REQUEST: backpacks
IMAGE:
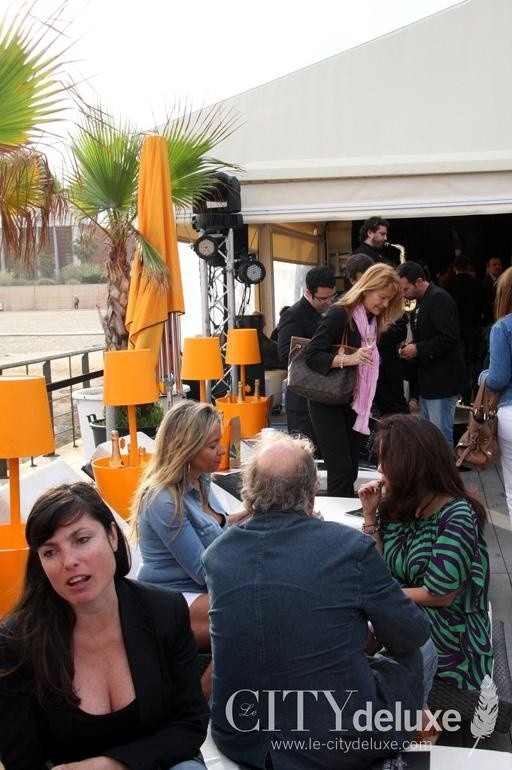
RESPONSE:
[285,334,362,406]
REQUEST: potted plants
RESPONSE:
[61,82,246,448]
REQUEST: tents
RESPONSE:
[124,136,186,396]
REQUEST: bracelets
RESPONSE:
[340,353,349,371]
[360,516,381,535]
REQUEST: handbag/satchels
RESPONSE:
[455,373,501,470]
[286,305,358,405]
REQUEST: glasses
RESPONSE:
[316,294,338,303]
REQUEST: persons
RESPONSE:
[0,478,212,770]
[197,428,440,769]
[443,255,485,416]
[476,264,512,536]
[483,255,504,325]
[304,261,404,498]
[131,397,232,704]
[346,254,410,415]
[270,306,289,343]
[277,265,336,459]
[357,216,390,264]
[395,262,459,460]
[250,311,277,358]
[357,412,495,689]
